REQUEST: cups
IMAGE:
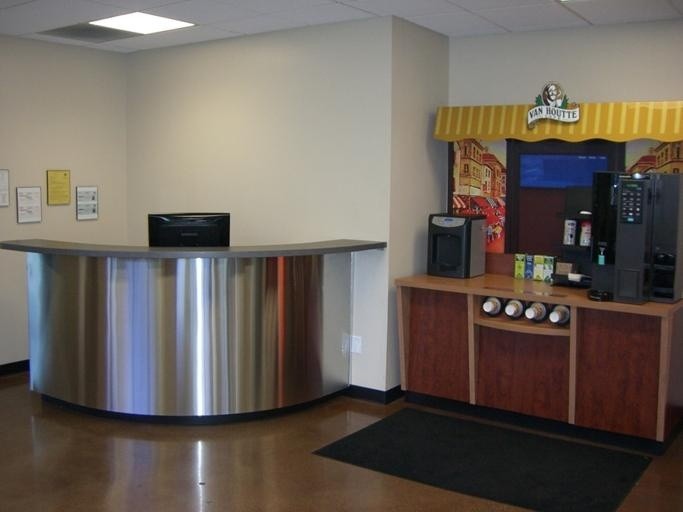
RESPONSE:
[483,298,570,326]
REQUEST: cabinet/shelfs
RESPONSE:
[395,271,682,444]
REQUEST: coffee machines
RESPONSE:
[428,214,489,277]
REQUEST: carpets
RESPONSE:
[309,406,654,512]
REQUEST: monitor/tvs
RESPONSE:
[148,214,232,247]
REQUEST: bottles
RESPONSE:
[578,219,591,247]
[563,218,576,246]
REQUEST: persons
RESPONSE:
[544,84,560,108]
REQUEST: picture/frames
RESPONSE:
[15,187,41,224]
[75,186,97,220]
[46,170,70,205]
[0,168,9,207]
